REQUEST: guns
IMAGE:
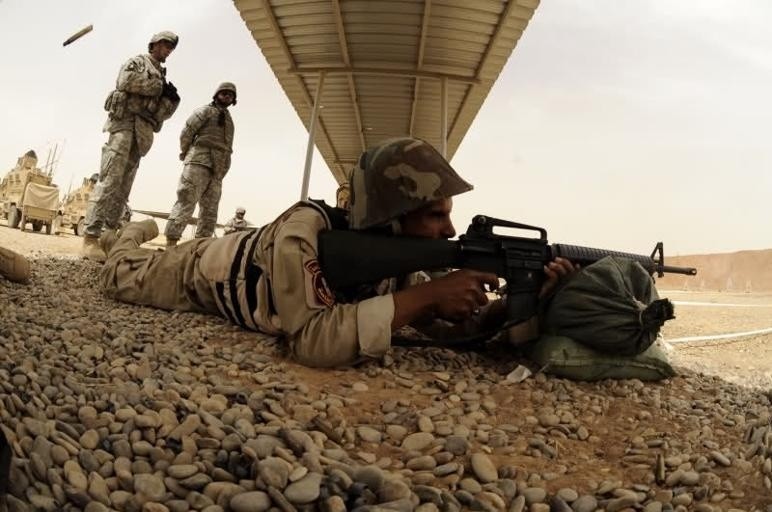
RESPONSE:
[313,213,698,325]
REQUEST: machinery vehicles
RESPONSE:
[62,174,130,237]
[1,150,60,233]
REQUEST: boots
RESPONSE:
[101,219,158,252]
[79,232,107,262]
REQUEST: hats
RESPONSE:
[236,207,245,214]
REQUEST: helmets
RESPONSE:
[147,30,179,53]
[349,137,473,231]
[213,82,236,99]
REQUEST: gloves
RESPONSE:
[179,152,185,161]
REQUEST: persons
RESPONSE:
[78,28,182,263]
[223,208,247,235]
[336,168,352,209]
[163,81,238,249]
[97,136,581,371]
